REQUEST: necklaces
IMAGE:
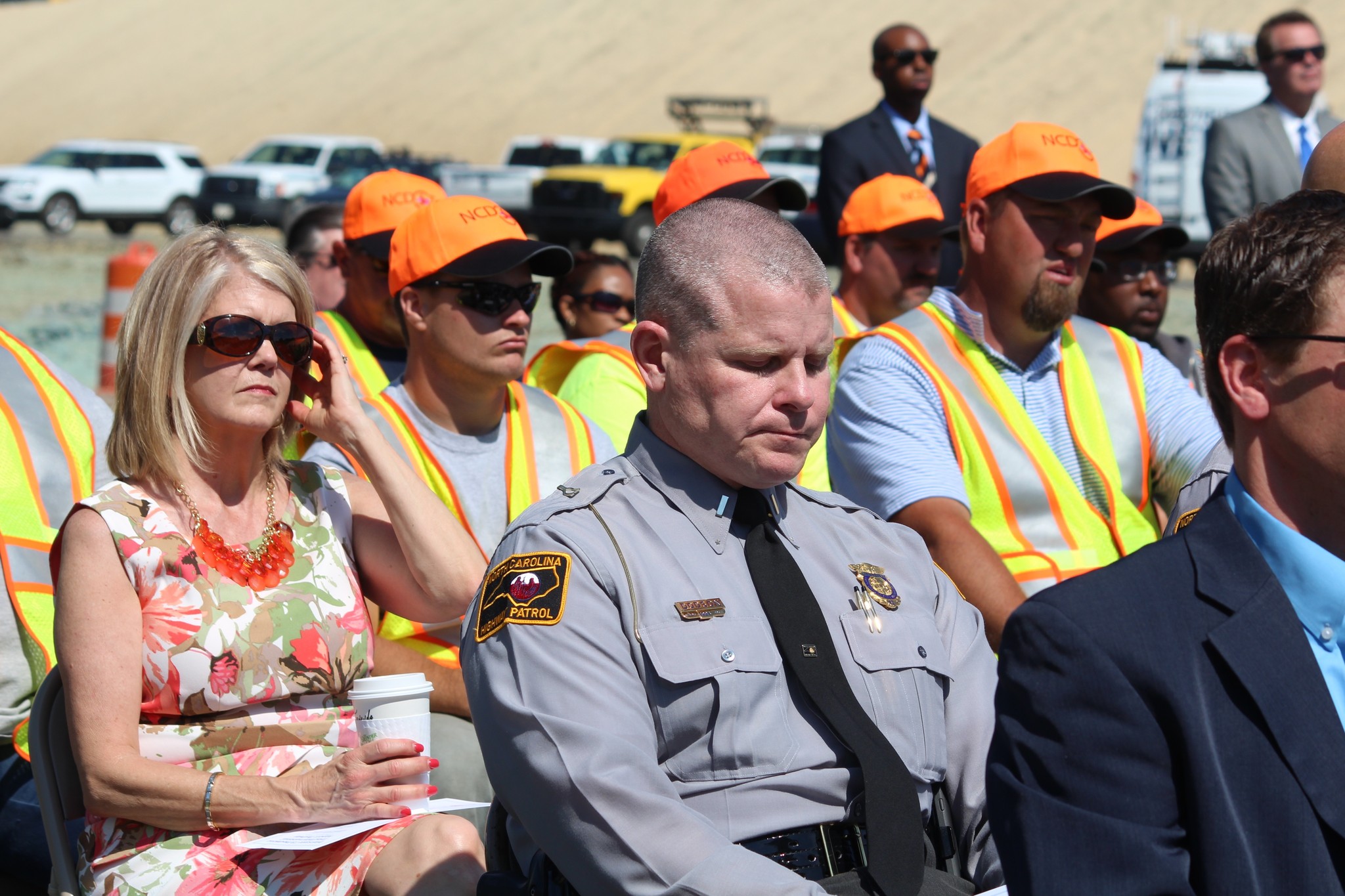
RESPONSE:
[165,458,295,591]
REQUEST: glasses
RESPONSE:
[886,50,936,67]
[352,242,388,270]
[1108,257,1177,283]
[422,277,541,316]
[189,315,315,363]
[1268,46,1323,64]
[577,291,635,316]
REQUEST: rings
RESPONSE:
[341,355,348,364]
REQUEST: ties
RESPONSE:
[736,488,924,896]
[907,129,937,191]
[1297,124,1312,169]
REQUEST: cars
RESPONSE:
[757,133,824,224]
[278,160,437,236]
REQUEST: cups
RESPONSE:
[347,673,436,814]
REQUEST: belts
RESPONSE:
[742,822,940,882]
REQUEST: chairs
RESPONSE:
[27,662,86,896]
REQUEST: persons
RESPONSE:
[48,227,488,896]
[459,197,999,896]
[284,9,1205,846]
[985,189,1345,896]
[1,333,120,896]
[1202,10,1345,235]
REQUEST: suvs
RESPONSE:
[531,131,756,257]
[198,132,389,229]
[1,141,208,237]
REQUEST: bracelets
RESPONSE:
[203,771,227,832]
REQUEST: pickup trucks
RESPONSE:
[432,138,606,235]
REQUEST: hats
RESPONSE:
[965,120,1136,220]
[1094,196,1189,256]
[343,166,447,248]
[837,172,957,239]
[387,194,573,298]
[651,141,807,227]
